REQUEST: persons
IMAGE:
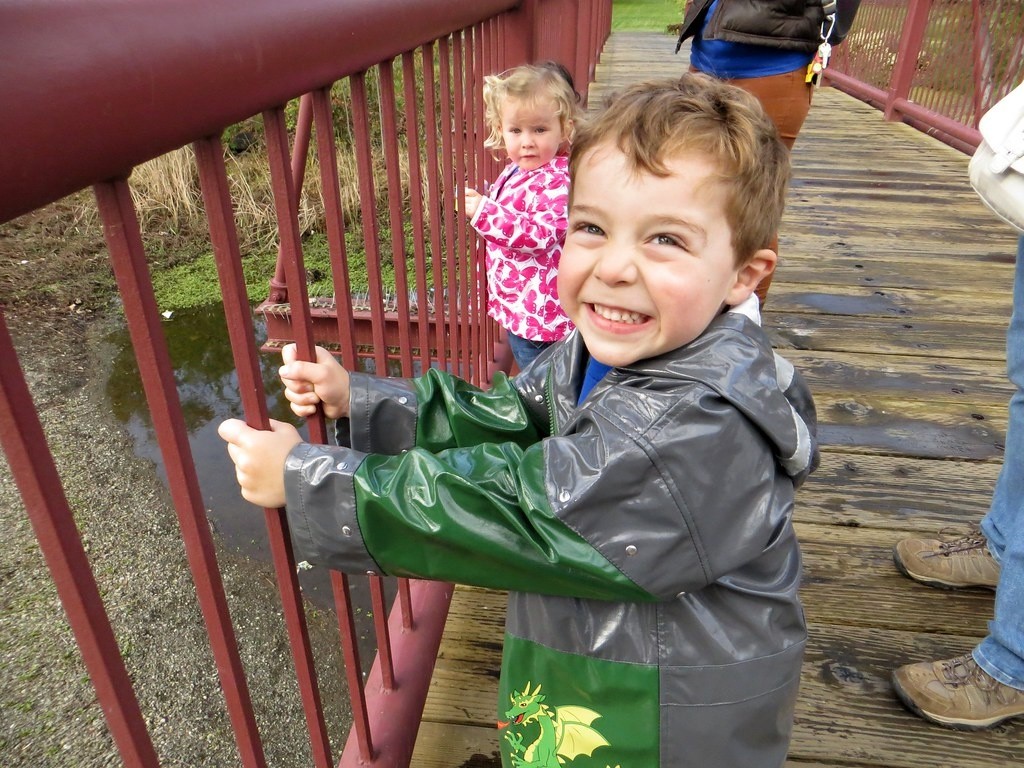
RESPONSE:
[455,63,574,371]
[675,0,861,314]
[890,81,1024,732]
[218,72,819,768]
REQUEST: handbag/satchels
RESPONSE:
[968,80,1024,233]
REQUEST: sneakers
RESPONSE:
[890,653,1024,732]
[893,521,1001,593]
